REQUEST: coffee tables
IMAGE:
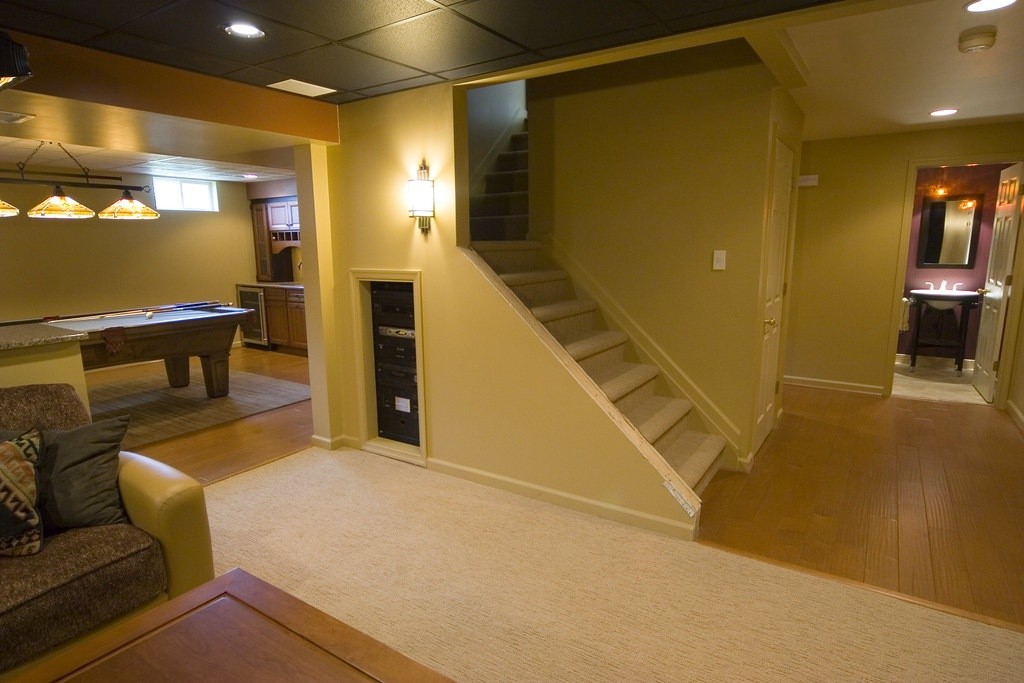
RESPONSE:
[1,567,457,683]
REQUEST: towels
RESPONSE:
[899,297,910,331]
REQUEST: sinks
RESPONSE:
[910,289,979,309]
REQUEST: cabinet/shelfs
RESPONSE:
[908,294,979,377]
[268,201,300,231]
[250,203,295,283]
[285,289,308,358]
[264,288,289,355]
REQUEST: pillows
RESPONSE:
[0,414,131,530]
[0,428,44,558]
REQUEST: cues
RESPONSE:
[48,299,233,324]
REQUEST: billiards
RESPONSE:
[146,311,154,318]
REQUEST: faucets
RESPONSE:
[939,280,947,290]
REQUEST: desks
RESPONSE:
[0,299,255,399]
[0,322,93,424]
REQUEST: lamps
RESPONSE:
[402,159,436,237]
[0,141,161,219]
[932,168,948,197]
[964,167,974,207]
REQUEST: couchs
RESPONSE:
[0,383,214,674]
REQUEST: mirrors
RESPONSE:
[915,193,985,270]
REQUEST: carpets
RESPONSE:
[85,362,312,452]
[204,447,1024,683]
